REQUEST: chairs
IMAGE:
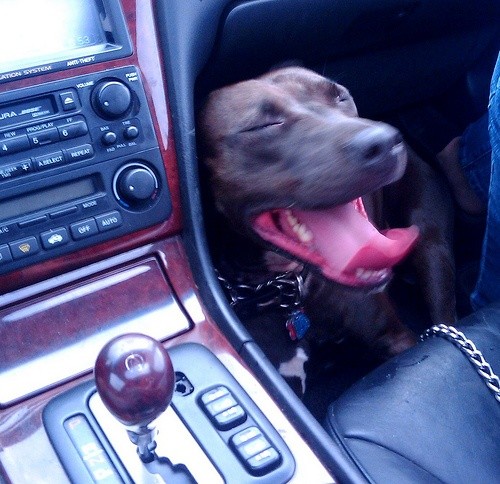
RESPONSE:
[330,303,499,484]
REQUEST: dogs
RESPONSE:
[196,66,465,358]
